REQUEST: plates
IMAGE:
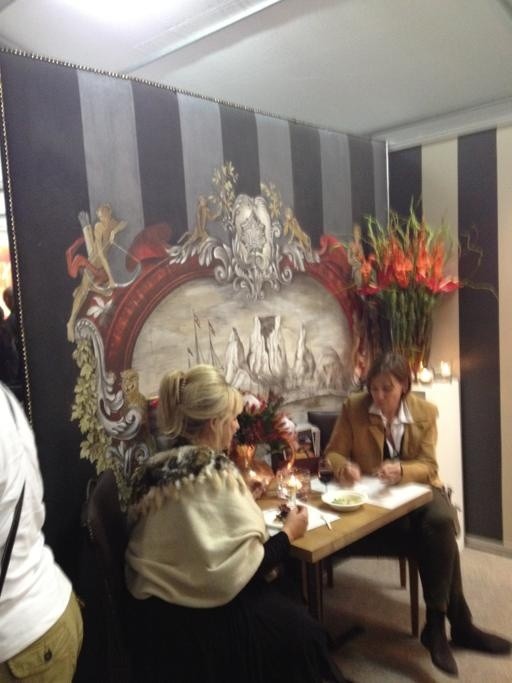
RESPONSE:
[262,506,320,528]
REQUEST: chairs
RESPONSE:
[307,409,418,637]
[78,467,129,683]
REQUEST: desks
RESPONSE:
[254,466,434,640]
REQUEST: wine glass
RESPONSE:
[318,458,334,491]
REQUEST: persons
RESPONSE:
[325,353,512,678]
[0,382,84,683]
[122,364,354,683]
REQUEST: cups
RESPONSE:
[275,468,311,499]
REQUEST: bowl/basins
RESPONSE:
[321,490,369,512]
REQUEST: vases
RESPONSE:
[230,444,275,500]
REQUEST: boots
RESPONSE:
[449,609,510,653]
[421,615,458,676]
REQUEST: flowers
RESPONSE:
[326,195,498,389]
[223,388,295,475]
